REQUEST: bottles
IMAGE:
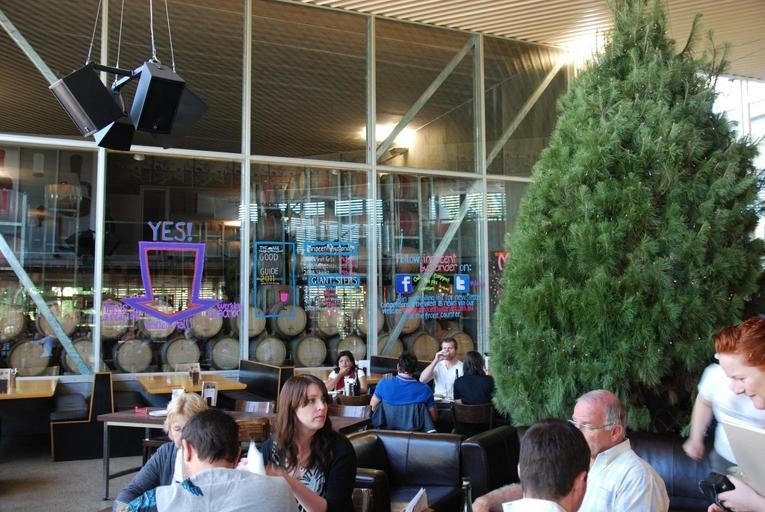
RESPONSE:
[189,365,199,386]
[0,372,7,394]
[461,482,472,512]
[345,381,359,396]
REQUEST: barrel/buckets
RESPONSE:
[0,301,476,375]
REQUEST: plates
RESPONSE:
[149,410,169,416]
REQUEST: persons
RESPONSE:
[111,392,210,512]
[705,316,765,512]
[237,374,358,512]
[369,351,439,423]
[125,409,301,512]
[503,418,592,511]
[418,337,464,402]
[682,360,764,476]
[471,390,671,512]
[325,350,368,395]
[453,351,495,405]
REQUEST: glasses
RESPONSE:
[567,420,613,431]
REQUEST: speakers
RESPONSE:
[130,61,187,136]
[93,86,136,152]
[47,63,124,140]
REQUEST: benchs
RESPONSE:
[0,355,509,512]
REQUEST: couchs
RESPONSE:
[459,422,737,512]
